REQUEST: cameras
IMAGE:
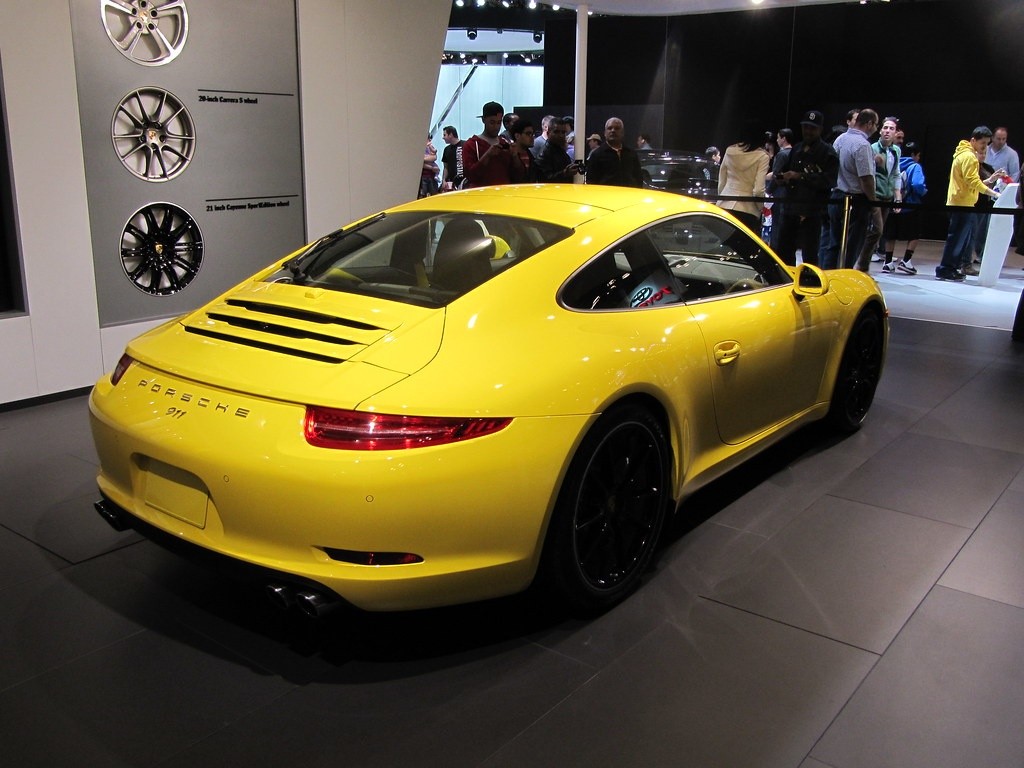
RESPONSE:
[775,172,784,179]
[574,160,585,175]
[499,142,510,149]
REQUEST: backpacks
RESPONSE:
[900,163,917,199]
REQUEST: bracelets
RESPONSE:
[987,178,993,183]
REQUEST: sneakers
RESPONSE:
[935,265,966,282]
[881,260,895,273]
[870,254,880,262]
[876,251,898,262]
[897,257,917,275]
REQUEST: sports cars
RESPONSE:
[88,183,889,615]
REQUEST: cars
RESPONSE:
[636,149,775,227]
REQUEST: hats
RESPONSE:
[586,133,601,141]
[800,110,824,129]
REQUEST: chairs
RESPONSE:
[429,217,492,291]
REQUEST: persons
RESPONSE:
[821,108,879,269]
[587,117,643,188]
[637,133,652,150]
[717,118,770,239]
[588,134,601,161]
[418,101,578,199]
[935,127,1024,282]
[772,110,840,265]
[831,106,1024,354]
[857,116,927,274]
[705,146,721,165]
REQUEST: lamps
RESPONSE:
[533,31,542,43]
[467,28,477,40]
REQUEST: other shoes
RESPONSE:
[961,265,980,276]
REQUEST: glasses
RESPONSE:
[553,129,567,135]
[521,131,536,138]
